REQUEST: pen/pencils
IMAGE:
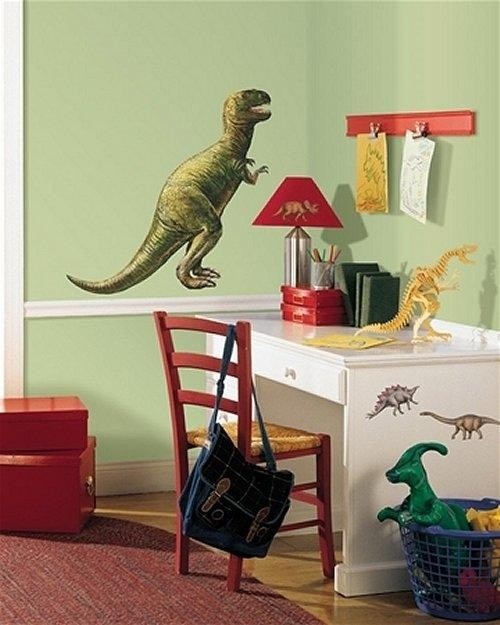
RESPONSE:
[305,243,342,287]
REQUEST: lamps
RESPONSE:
[252,175,343,287]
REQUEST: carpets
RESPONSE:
[1,516,326,625]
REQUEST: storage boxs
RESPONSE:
[0,397,96,536]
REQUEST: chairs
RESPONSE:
[150,309,332,591]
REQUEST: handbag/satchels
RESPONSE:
[177,327,295,558]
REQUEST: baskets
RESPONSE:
[396,498,499,620]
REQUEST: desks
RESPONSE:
[196,311,500,596]
